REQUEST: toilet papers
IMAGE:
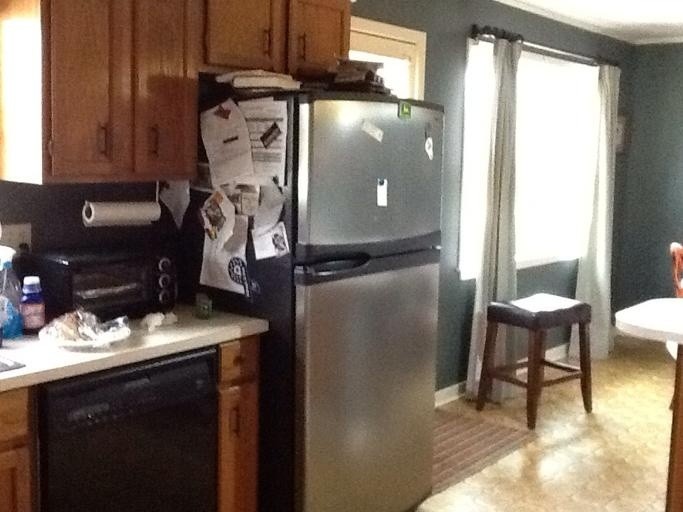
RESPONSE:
[82,201,162,228]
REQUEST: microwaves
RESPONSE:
[20,244,177,326]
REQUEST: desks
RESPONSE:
[615,297,683,511]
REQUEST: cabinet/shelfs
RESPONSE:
[0,0,202,185]
[202,0,347,78]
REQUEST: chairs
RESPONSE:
[669,242,683,299]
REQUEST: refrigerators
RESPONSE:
[194,87,447,511]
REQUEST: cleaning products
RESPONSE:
[0,245,24,339]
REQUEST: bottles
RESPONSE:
[19,276,45,337]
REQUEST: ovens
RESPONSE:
[36,349,218,511]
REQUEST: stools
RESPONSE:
[476,291,592,431]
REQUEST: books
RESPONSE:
[231,75,303,91]
[214,69,293,85]
[323,59,391,95]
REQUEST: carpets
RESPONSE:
[431,406,540,496]
